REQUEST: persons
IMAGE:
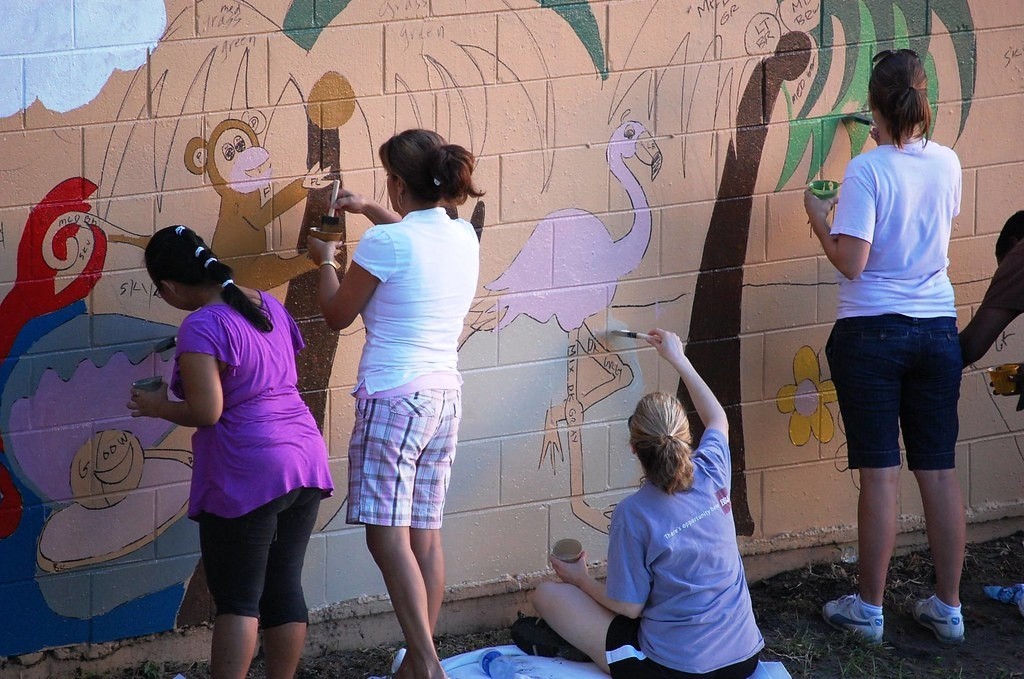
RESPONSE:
[531,328,765,679]
[127,225,335,678]
[306,129,486,679]
[804,49,966,649]
[957,210,1024,411]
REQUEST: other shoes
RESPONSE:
[511,616,593,662]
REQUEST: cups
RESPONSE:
[309,226,343,242]
[550,538,583,563]
[131,376,163,392]
[987,364,1019,394]
[807,180,840,199]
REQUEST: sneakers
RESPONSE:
[821,593,884,642]
[912,593,965,645]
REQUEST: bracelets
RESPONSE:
[318,260,337,270]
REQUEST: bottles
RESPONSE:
[478,648,516,679]
[983,585,1022,606]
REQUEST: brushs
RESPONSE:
[320,180,341,232]
[155,337,178,352]
[611,329,661,341]
[846,113,878,127]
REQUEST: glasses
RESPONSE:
[866,48,921,101]
